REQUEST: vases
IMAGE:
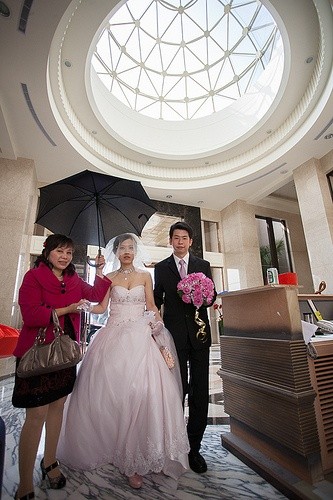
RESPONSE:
[218,320,224,335]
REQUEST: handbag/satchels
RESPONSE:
[17,310,83,378]
[158,335,175,369]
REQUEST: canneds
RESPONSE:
[267,268,279,285]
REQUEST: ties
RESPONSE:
[179,260,186,279]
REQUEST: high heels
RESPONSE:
[14,490,35,500]
[40,457,67,489]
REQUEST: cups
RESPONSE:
[302,313,314,325]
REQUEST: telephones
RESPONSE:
[317,319,333,333]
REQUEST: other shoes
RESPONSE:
[129,474,143,489]
[188,450,208,473]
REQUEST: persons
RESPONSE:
[56,232,191,489]
[11,234,112,500]
[152,221,218,473]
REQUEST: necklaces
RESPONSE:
[57,275,63,278]
[117,266,135,280]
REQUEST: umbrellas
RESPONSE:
[33,170,157,268]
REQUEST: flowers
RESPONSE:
[176,272,214,307]
[213,302,223,320]
